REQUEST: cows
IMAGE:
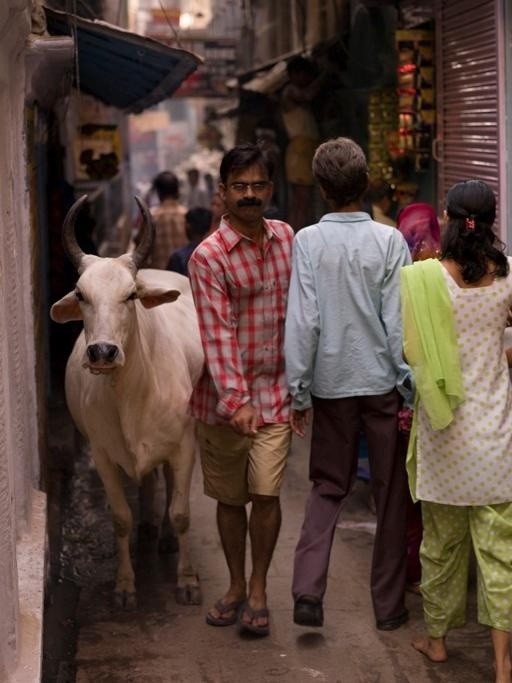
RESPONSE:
[50,193,205,611]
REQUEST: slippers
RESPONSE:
[207,599,323,635]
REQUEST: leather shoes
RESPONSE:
[377,613,408,630]
